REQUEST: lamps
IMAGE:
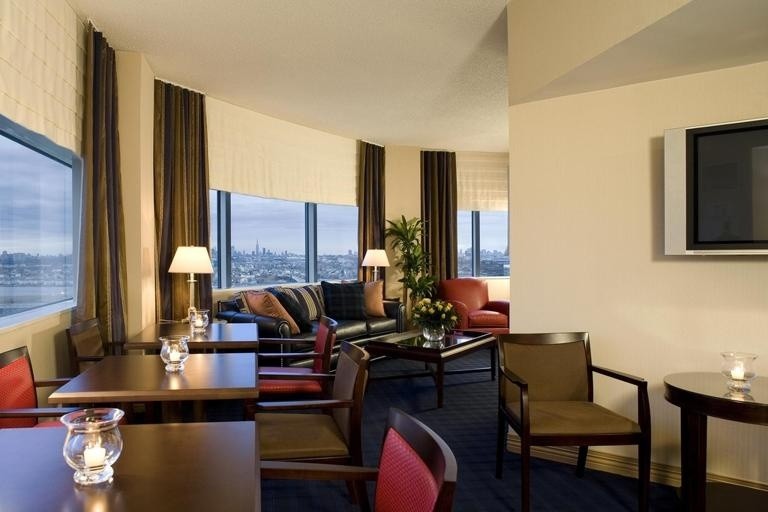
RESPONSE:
[167,246,215,322]
[361,249,390,281]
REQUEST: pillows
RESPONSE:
[233,281,385,333]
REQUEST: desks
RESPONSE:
[663,373,768,512]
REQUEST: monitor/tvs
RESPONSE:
[664,117,768,256]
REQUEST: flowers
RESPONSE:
[412,298,462,329]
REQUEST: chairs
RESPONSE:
[437,278,510,341]
[497,332,652,512]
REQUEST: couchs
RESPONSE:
[218,281,408,374]
